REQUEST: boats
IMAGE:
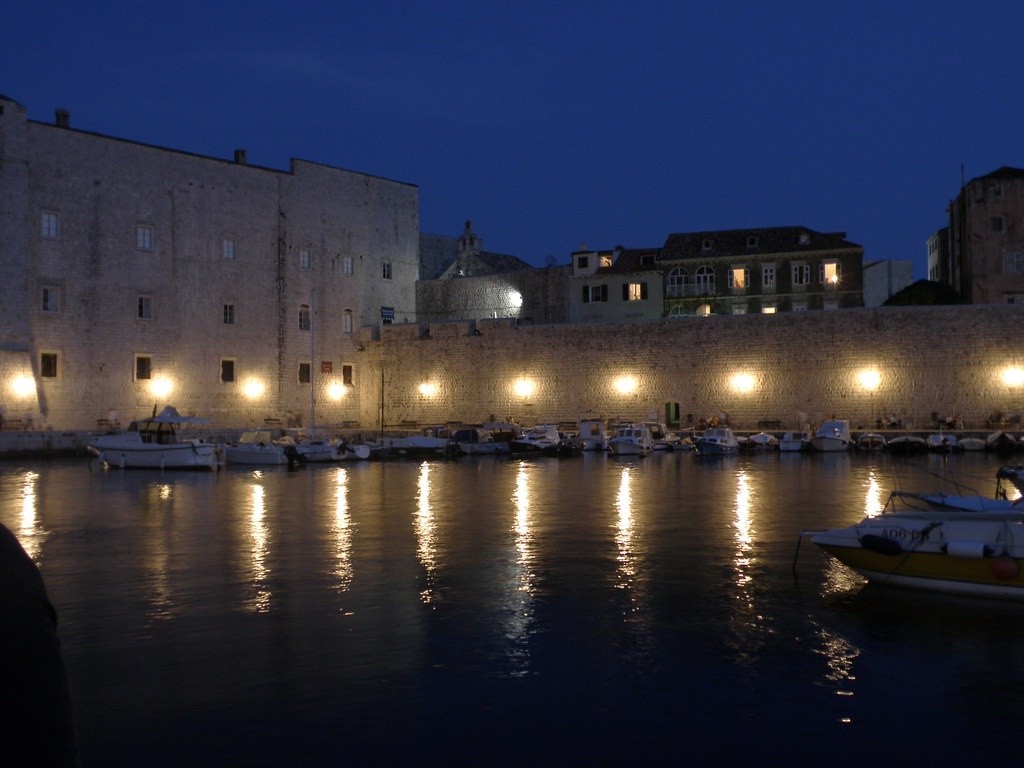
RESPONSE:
[86,405,226,469]
[799,465,1024,642]
[777,431,811,453]
[224,418,778,465]
[810,420,1024,457]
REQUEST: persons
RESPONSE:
[882,413,898,429]
[946,416,963,429]
[108,408,117,424]
[287,409,303,428]
[23,407,35,431]
[988,412,1005,430]
[707,415,717,428]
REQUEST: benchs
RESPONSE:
[559,421,577,429]
[97,419,121,430]
[936,420,957,429]
[758,421,781,429]
[400,421,417,428]
[878,420,902,429]
[445,421,463,426]
[986,420,1012,428]
[342,420,360,428]
[264,419,282,426]
[1,420,24,431]
